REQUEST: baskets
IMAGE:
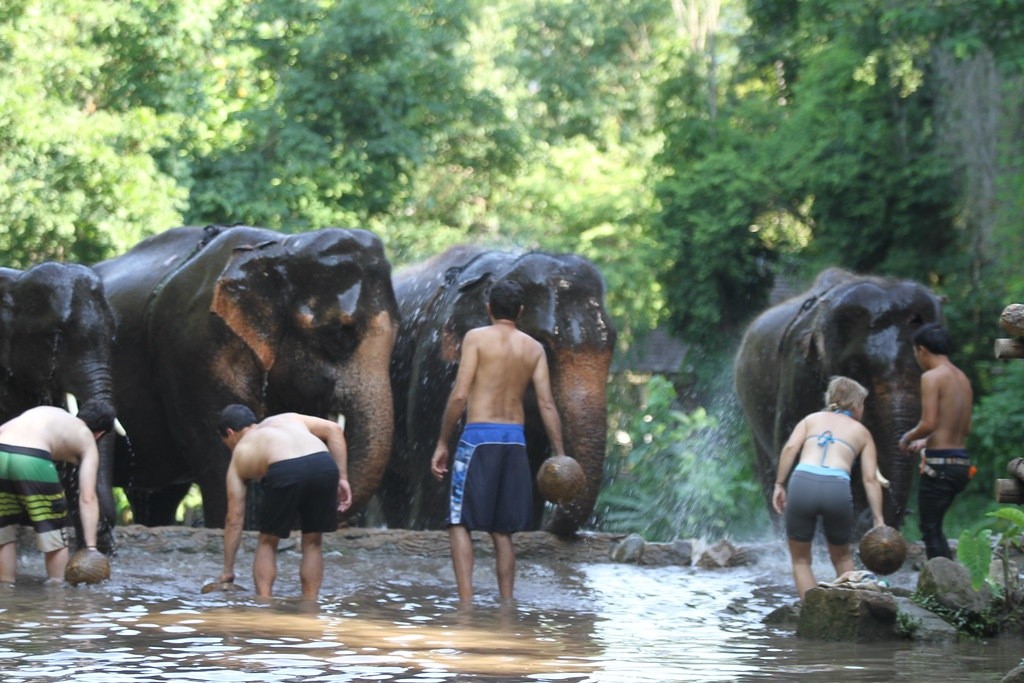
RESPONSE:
[201,581,247,595]
[536,457,586,505]
[856,526,906,576]
[64,548,110,586]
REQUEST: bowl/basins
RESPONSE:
[537,456,586,503]
[65,550,110,585]
[201,583,247,593]
[858,526,906,574]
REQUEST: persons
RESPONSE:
[432,279,564,603]
[773,377,885,601]
[218,405,352,596]
[0,398,115,583]
[899,323,972,564]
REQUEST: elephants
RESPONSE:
[1,262,128,559]
[361,243,617,534]
[735,265,943,546]
[94,222,404,528]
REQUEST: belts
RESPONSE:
[920,456,970,479]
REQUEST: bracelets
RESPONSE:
[85,546,97,548]
[902,436,910,445]
[775,481,784,485]
